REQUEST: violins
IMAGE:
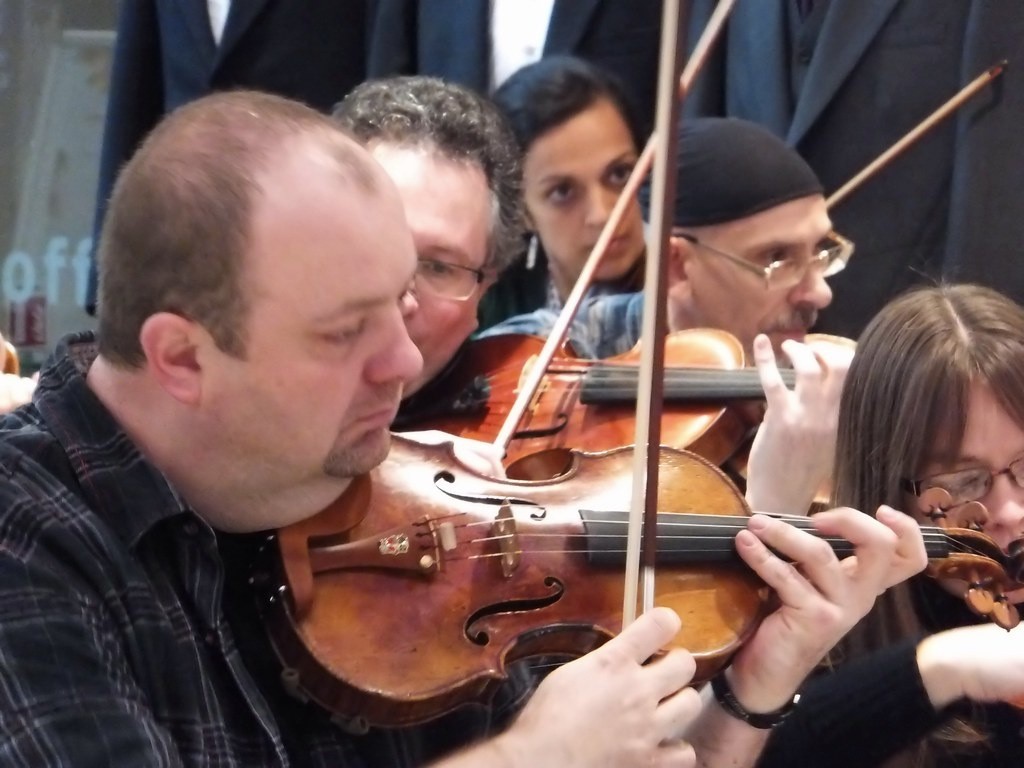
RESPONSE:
[730,333,858,517]
[403,327,799,481]
[247,431,1024,730]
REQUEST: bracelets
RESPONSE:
[709,670,805,729]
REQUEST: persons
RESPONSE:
[0,95,926,768]
[490,53,653,305]
[469,120,844,376]
[86,0,1024,336]
[326,76,857,516]
[754,284,1024,768]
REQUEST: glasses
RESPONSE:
[688,227,855,287]
[901,457,1024,509]
[408,258,484,303]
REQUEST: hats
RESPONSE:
[645,115,823,226]
